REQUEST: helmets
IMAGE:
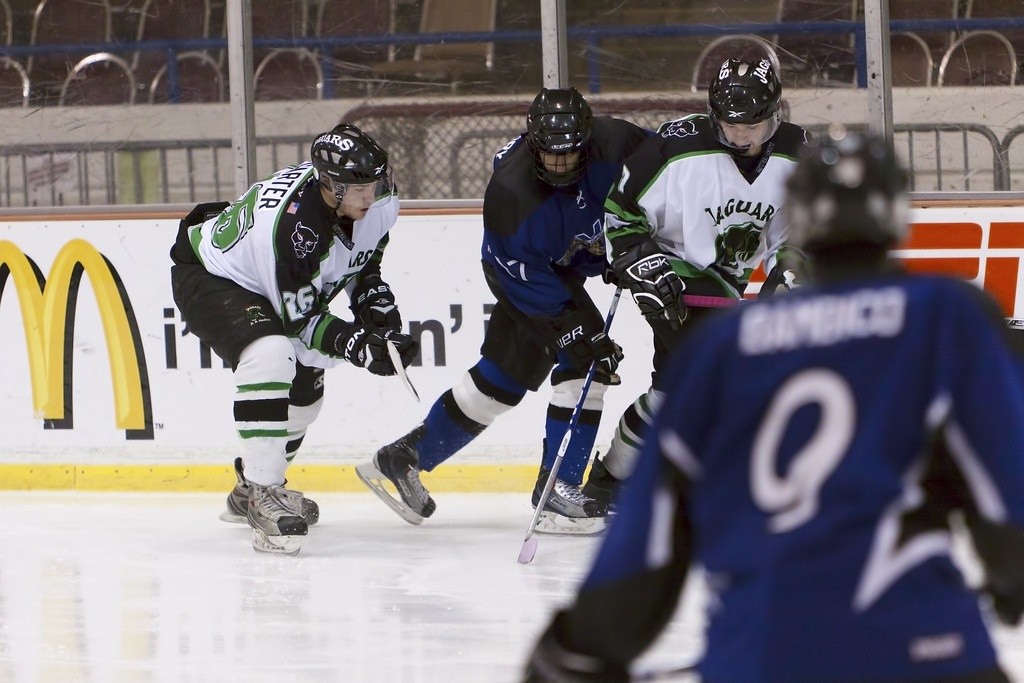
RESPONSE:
[709,56,782,127]
[525,87,592,186]
[311,124,389,186]
[785,129,911,250]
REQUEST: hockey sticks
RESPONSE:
[679,291,1024,330]
[514,278,627,568]
[381,336,425,406]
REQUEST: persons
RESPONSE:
[355,37,1023,683]
[170,122,421,557]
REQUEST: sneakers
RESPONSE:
[246,478,308,557]
[581,450,623,523]
[355,421,436,525]
[531,438,606,534]
[219,457,319,525]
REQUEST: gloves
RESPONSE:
[545,317,624,373]
[349,276,402,333]
[966,508,1024,624]
[611,239,688,331]
[758,265,805,300]
[524,611,630,683]
[334,322,419,376]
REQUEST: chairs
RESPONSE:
[0,0,1023,107]
